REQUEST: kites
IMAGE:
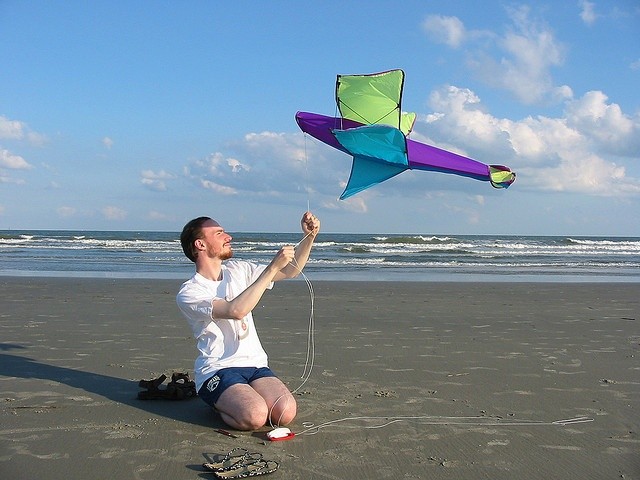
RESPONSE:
[295,68,518,201]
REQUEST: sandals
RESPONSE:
[137,374,191,401]
[168,372,196,399]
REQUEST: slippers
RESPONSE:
[203,447,262,470]
[214,460,280,479]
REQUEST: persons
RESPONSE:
[176,209,322,431]
